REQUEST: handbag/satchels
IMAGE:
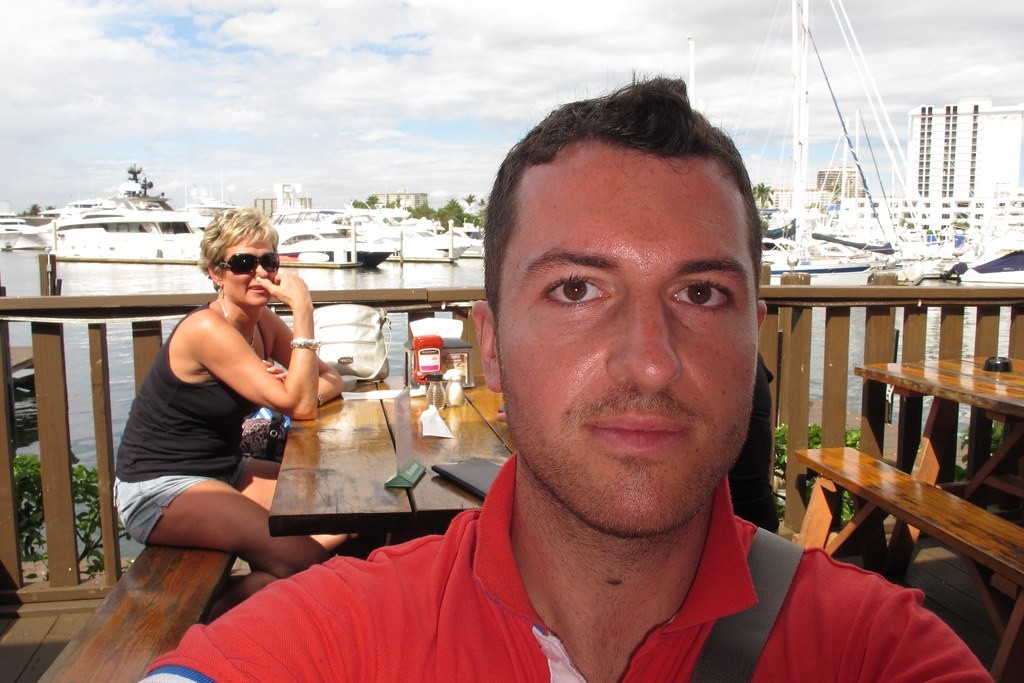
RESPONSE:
[312,302,393,382]
[239,413,287,462]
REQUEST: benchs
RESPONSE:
[37,545,237,683]
[795,447,1024,683]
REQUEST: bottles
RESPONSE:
[427,374,445,409]
[444,369,465,407]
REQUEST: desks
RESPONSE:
[268,374,514,559]
[854,356,1024,592]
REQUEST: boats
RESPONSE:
[0,183,487,270]
[681,1,1023,284]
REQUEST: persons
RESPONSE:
[728,350,781,533]
[135,76,995,683]
[112,207,348,626]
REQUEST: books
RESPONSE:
[430,460,504,501]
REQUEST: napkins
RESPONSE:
[420,404,452,438]
[409,317,464,340]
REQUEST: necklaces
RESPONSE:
[215,299,255,348]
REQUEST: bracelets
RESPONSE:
[317,393,322,408]
[289,338,319,351]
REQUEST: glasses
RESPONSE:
[215,252,282,277]
